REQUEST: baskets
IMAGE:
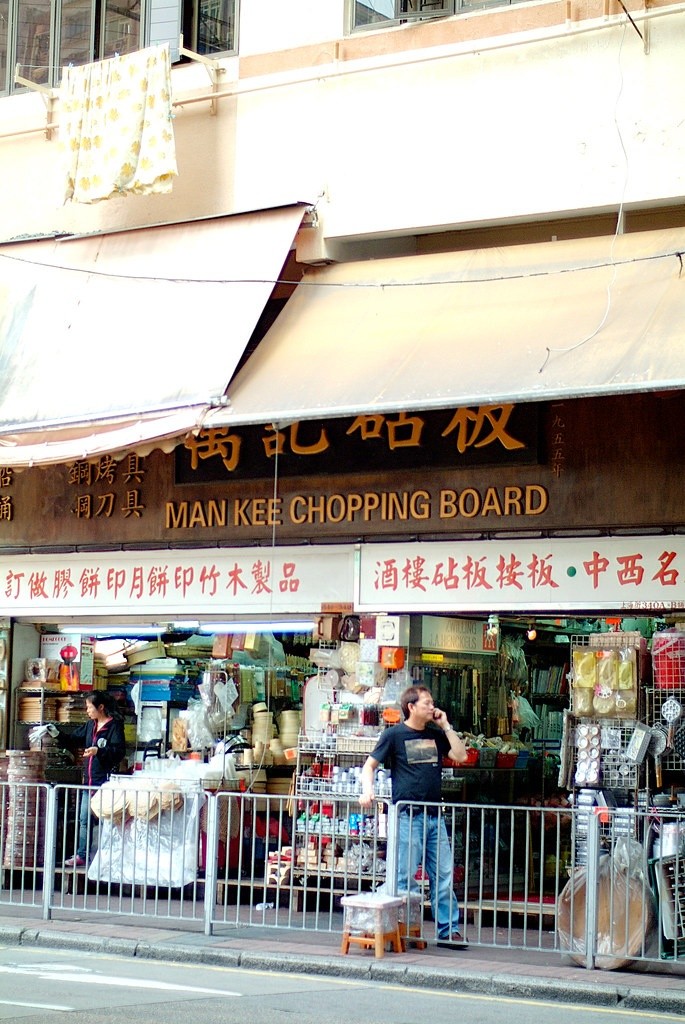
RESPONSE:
[442,747,530,769]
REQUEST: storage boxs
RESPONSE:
[496,752,518,768]
[24,657,61,681]
[515,756,530,768]
[479,748,498,767]
[440,747,479,767]
[40,634,95,691]
[356,662,389,687]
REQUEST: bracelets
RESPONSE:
[443,725,454,732]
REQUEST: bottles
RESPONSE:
[499,741,510,754]
[469,733,485,749]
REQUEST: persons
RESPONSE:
[358,686,470,949]
[48,691,126,867]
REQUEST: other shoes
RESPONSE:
[65,854,86,865]
[437,930,469,950]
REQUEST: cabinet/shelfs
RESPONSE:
[134,701,189,771]
[13,687,94,787]
[290,728,388,911]
[236,764,296,880]
[501,621,597,741]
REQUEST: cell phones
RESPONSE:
[433,713,441,720]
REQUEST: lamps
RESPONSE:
[198,619,315,632]
[527,624,537,640]
[487,615,499,636]
[57,623,174,634]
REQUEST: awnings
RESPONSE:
[200,225,685,428]
[0,201,318,436]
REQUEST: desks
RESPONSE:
[454,769,529,881]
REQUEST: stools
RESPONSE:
[340,893,402,958]
[367,891,426,950]
